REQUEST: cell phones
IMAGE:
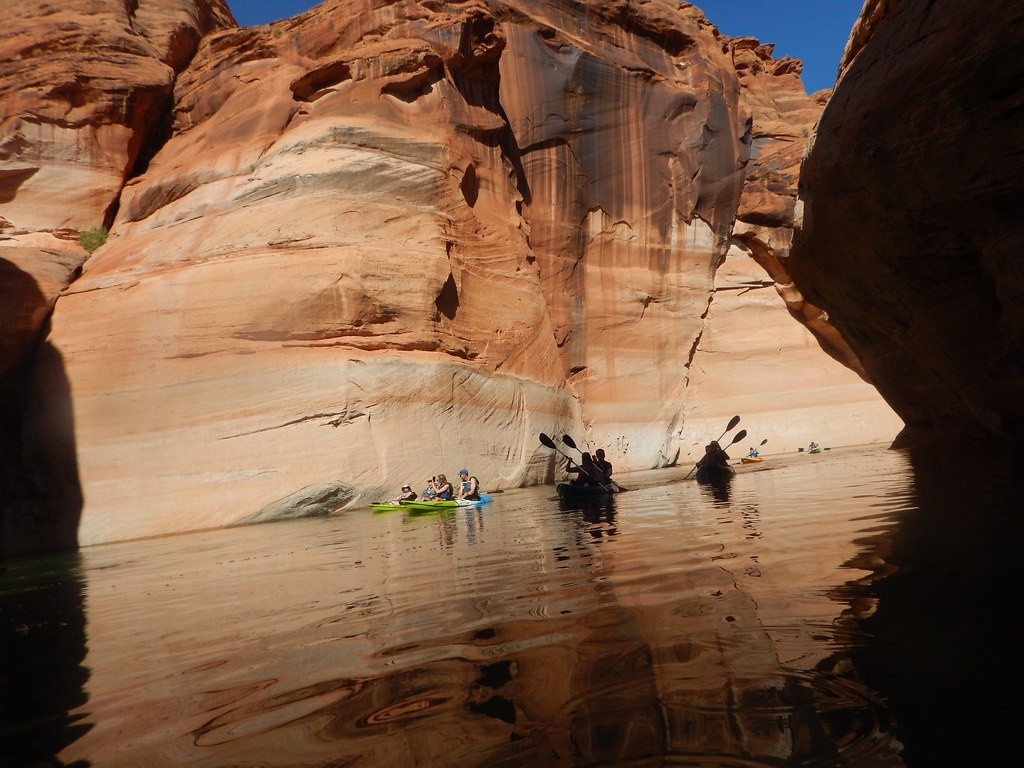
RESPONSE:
[433,477,435,482]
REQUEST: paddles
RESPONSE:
[539,433,630,493]
[479,490,504,495]
[690,429,747,479]
[683,415,741,481]
[756,438,769,449]
[371,499,398,504]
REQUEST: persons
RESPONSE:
[397,485,418,502]
[749,447,759,459]
[695,441,730,469]
[421,480,436,501]
[432,474,453,501]
[809,442,816,450]
[458,469,480,500]
[565,449,612,485]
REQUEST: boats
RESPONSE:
[808,448,821,453]
[369,503,405,513]
[401,494,493,516]
[556,482,620,498]
[741,456,764,464]
[696,463,735,480]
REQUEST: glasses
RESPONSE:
[460,474,467,477]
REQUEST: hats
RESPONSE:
[458,470,469,475]
[401,485,412,491]
[428,480,433,482]
